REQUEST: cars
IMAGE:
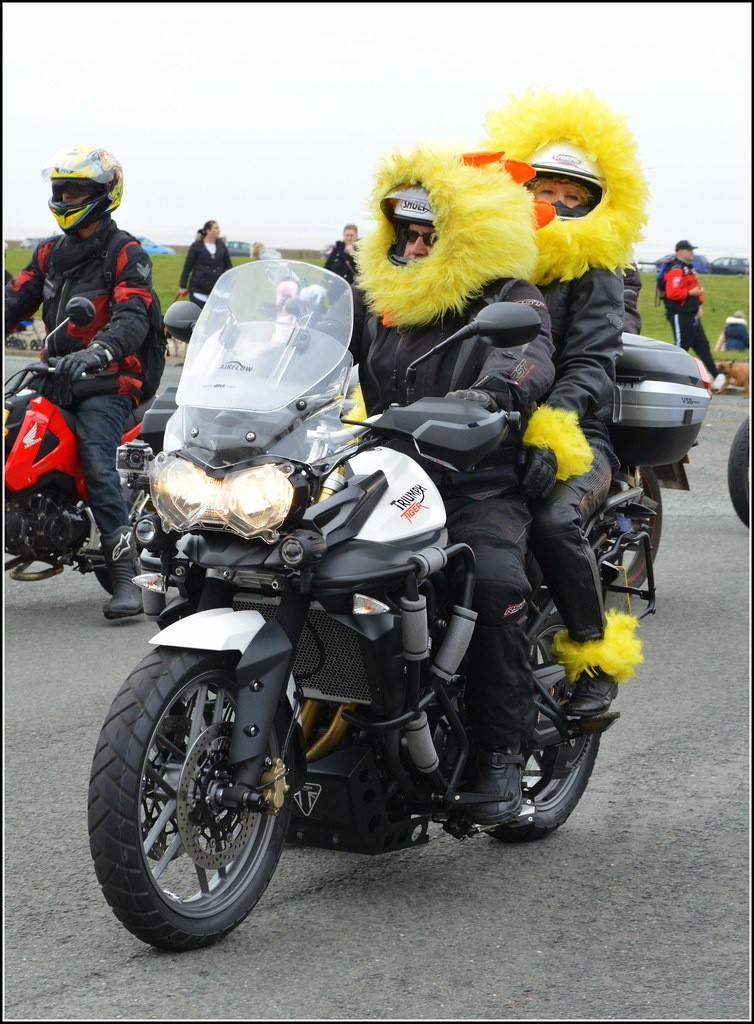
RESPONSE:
[137,238,176,254]
[710,257,749,277]
[259,249,281,260]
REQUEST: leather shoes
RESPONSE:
[563,665,619,716]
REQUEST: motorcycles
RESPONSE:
[728,417,749,529]
[86,261,711,953]
[3,292,178,578]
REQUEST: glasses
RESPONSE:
[402,229,437,246]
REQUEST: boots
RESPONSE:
[99,525,146,619]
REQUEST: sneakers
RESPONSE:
[462,739,525,824]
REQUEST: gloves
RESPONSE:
[444,373,512,409]
[516,438,558,499]
[55,343,109,382]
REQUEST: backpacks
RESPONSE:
[654,260,676,299]
[52,226,169,401]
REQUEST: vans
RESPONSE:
[689,255,709,273]
[224,241,252,257]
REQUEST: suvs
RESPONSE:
[320,244,334,258]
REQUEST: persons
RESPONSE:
[188,141,556,826]
[663,240,719,378]
[4,144,153,621]
[251,242,301,337]
[179,220,235,337]
[725,309,749,350]
[323,223,362,307]
[483,83,652,718]
[623,252,642,335]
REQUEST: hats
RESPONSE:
[484,104,644,284]
[676,240,697,253]
[355,149,559,328]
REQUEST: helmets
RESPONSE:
[526,138,607,222]
[379,181,439,267]
[43,150,123,232]
